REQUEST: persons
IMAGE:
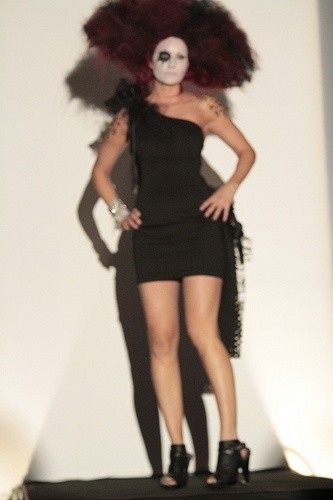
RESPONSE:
[83,1,260,489]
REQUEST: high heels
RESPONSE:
[161,444,193,489]
[207,438,251,489]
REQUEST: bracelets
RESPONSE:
[108,198,131,226]
